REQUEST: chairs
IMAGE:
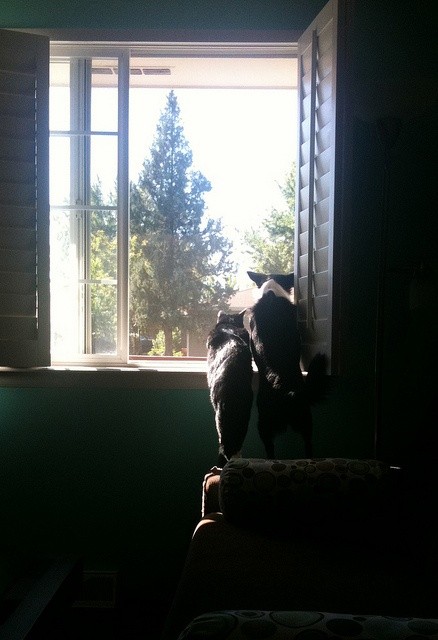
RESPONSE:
[157,456,438,638]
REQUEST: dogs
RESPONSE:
[247,270,336,459]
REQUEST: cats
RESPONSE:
[206,307,253,473]
